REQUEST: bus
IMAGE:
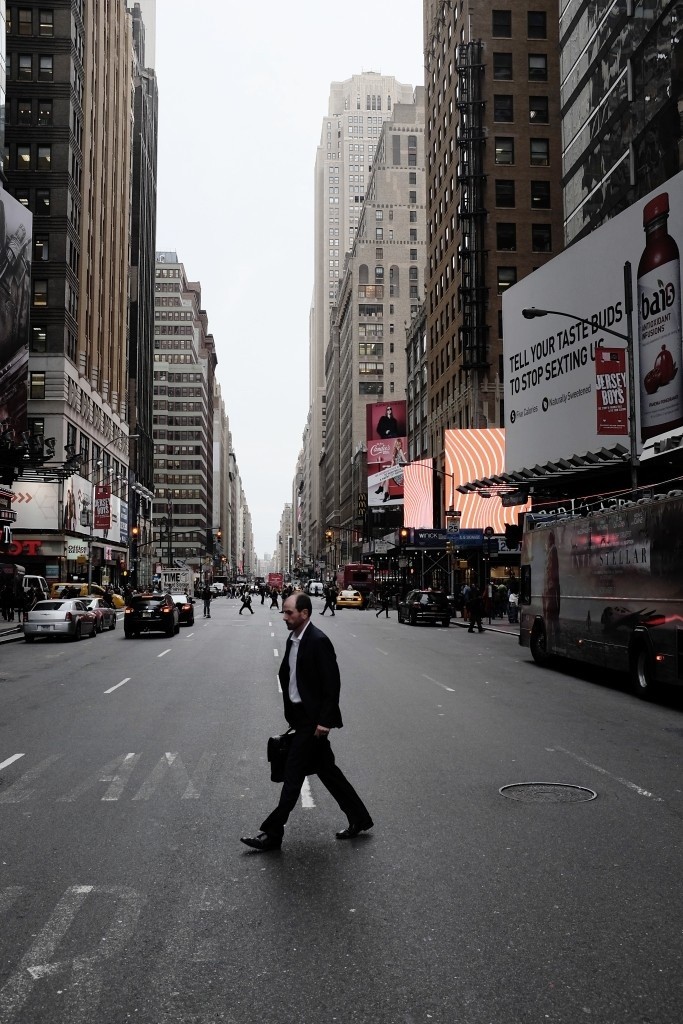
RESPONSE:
[237,575,247,590]
[254,576,264,588]
[269,573,282,587]
[336,563,374,594]
[213,576,228,596]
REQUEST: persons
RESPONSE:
[239,594,374,852]
[201,581,340,619]
[376,405,400,438]
[364,579,518,633]
[375,439,408,504]
[0,577,162,623]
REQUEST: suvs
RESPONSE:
[398,588,451,628]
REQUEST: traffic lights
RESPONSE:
[223,558,226,565]
[326,531,332,542]
[132,528,138,534]
[218,532,221,542]
[399,528,409,545]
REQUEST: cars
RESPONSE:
[335,585,363,610]
[170,593,196,627]
[23,598,96,642]
[303,579,323,596]
[72,597,116,633]
[123,593,179,636]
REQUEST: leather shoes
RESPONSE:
[240,834,283,849]
[336,818,374,839]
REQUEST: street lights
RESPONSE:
[88,432,143,590]
[522,306,639,498]
[398,460,456,618]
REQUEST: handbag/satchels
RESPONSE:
[267,727,317,783]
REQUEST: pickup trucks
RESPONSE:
[50,582,125,609]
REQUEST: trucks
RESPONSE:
[0,563,50,612]
[520,492,683,710]
